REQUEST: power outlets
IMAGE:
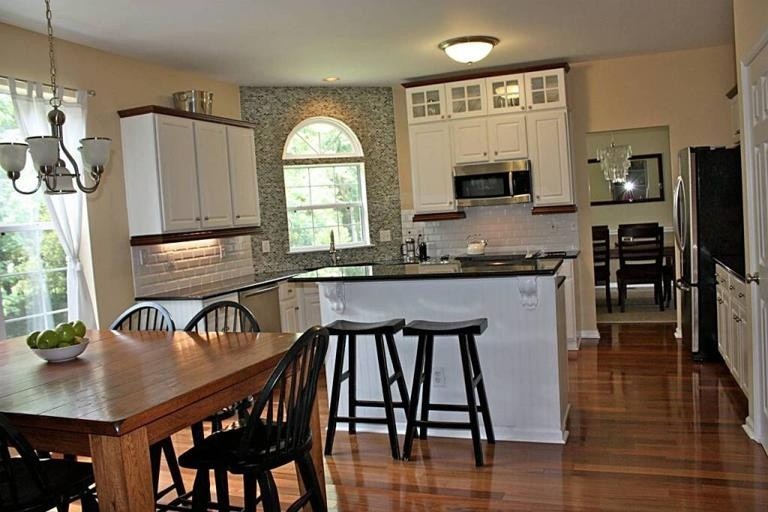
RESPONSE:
[260,240,270,253]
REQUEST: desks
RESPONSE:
[593,246,676,309]
[135,269,305,331]
[288,256,568,441]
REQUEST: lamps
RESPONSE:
[595,143,632,186]
[0,1,112,194]
[495,83,522,105]
[436,35,500,65]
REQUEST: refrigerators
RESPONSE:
[672,146,741,364]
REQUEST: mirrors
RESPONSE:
[587,154,665,205]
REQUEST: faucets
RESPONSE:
[329,243,342,265]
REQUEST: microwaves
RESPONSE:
[453,159,533,207]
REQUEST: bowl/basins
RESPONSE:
[31,338,90,364]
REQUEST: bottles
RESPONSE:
[406,239,415,262]
[420,228,427,261]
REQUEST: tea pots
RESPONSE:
[465,234,489,254]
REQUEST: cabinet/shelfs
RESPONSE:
[444,64,529,218]
[401,78,454,221]
[117,96,233,246]
[203,291,241,332]
[727,83,742,143]
[225,115,261,247]
[716,260,752,395]
[526,64,575,214]
[239,282,281,332]
[279,281,321,332]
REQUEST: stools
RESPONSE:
[402,319,495,468]
[323,319,420,458]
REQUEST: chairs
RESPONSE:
[104,301,188,507]
[178,327,332,511]
[592,227,610,316]
[0,411,97,512]
[617,228,664,312]
[182,301,260,512]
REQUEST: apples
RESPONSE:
[55,323,74,342]
[67,320,86,338]
[27,331,40,349]
[36,330,58,349]
[59,343,71,348]
